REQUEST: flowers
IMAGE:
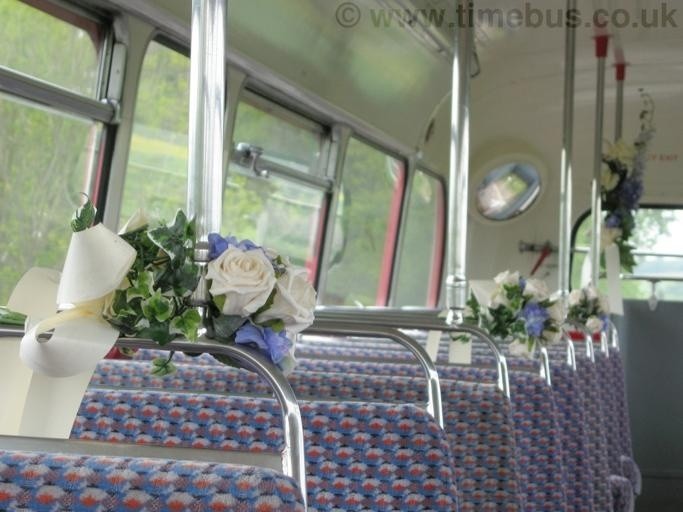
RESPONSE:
[464,273,610,349]
[594,85,655,224]
[102,206,316,380]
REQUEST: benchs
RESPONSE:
[2,313,640,512]
